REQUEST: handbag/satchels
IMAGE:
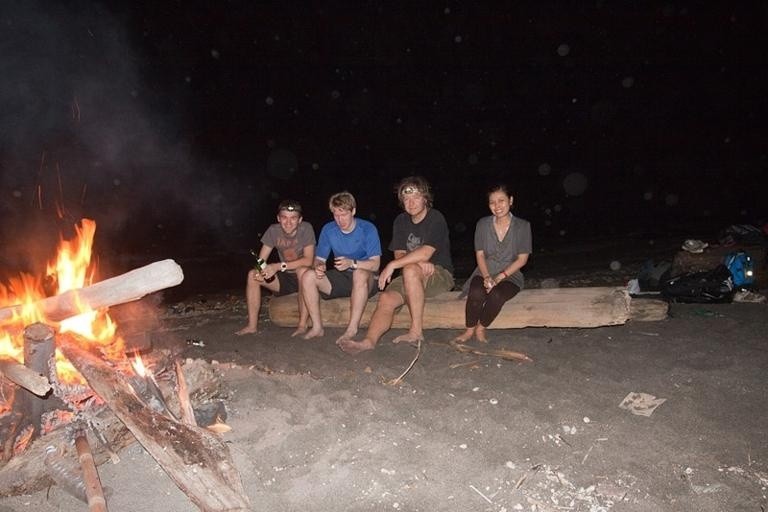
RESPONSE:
[661,264,737,305]
[724,248,755,286]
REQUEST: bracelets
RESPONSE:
[502,272,509,278]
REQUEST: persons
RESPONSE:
[301,193,382,344]
[234,200,316,337]
[456,185,532,343]
[339,177,456,352]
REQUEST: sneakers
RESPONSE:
[733,290,766,303]
[680,224,762,254]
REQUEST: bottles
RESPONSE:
[743,257,753,279]
[44,444,113,504]
[247,247,275,283]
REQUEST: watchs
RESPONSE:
[280,262,288,272]
[352,259,357,271]
